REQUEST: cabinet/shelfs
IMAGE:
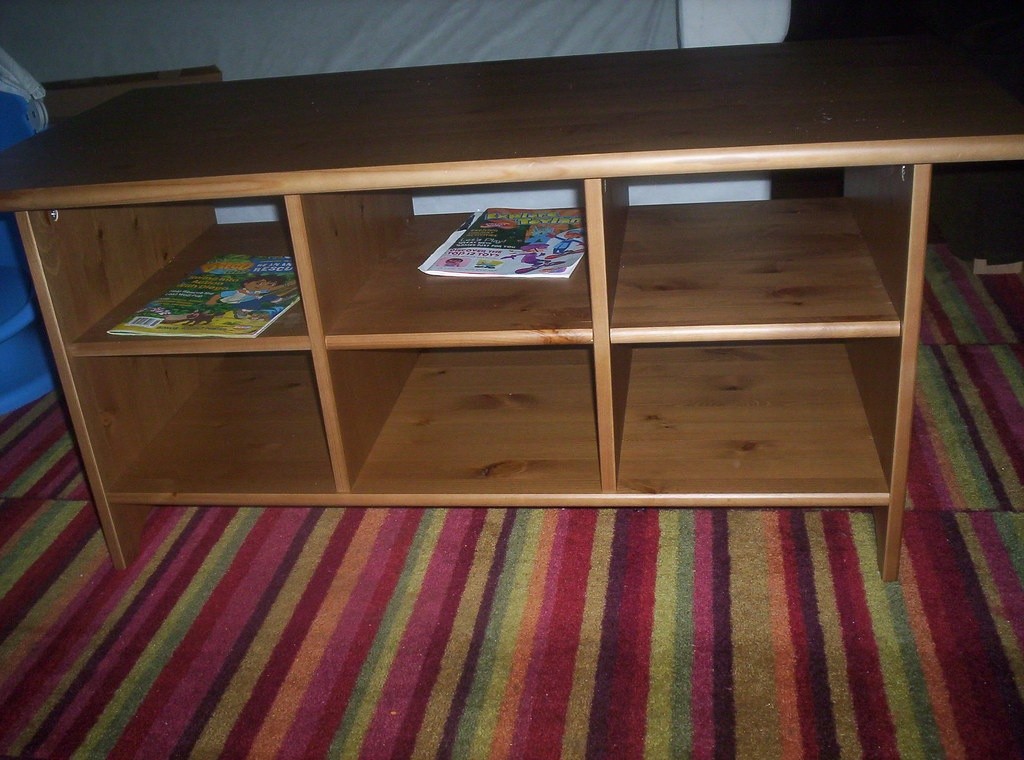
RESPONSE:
[0,26,1024,585]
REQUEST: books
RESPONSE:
[106,256,301,339]
[417,207,585,279]
